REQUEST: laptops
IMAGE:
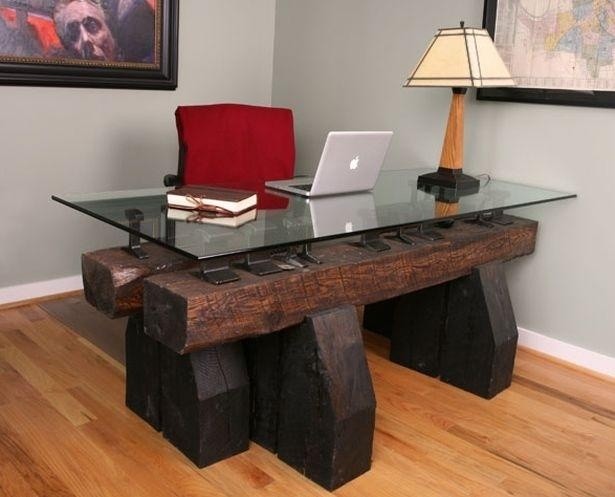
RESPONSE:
[265,131,394,198]
[265,188,378,239]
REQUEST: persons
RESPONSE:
[52,1,120,62]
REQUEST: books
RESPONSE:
[166,184,259,216]
[164,205,258,229]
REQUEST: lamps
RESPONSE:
[401,21,520,190]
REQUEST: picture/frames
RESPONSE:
[0,1,181,90]
[475,0,615,111]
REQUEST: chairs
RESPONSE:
[163,103,297,192]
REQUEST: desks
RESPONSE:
[49,166,578,491]
